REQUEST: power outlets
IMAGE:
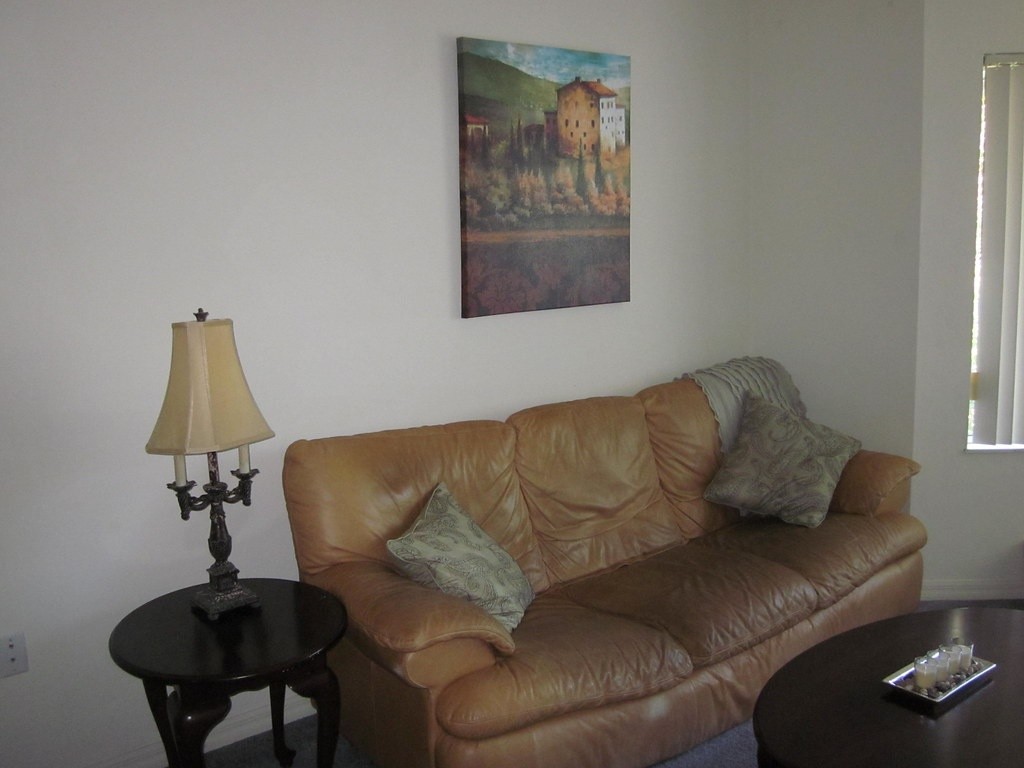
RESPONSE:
[0,631,30,679]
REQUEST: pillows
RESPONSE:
[382,480,536,636]
[703,389,862,529]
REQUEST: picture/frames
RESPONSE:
[455,36,632,318]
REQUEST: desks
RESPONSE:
[109,578,347,767]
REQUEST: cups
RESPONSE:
[927,651,949,682]
[914,657,936,689]
[940,644,962,676]
[950,637,974,669]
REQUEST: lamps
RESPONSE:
[145,309,276,622]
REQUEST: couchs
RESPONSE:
[281,357,928,768]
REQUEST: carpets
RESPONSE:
[655,717,758,768]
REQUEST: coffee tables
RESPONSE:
[751,605,1022,768]
